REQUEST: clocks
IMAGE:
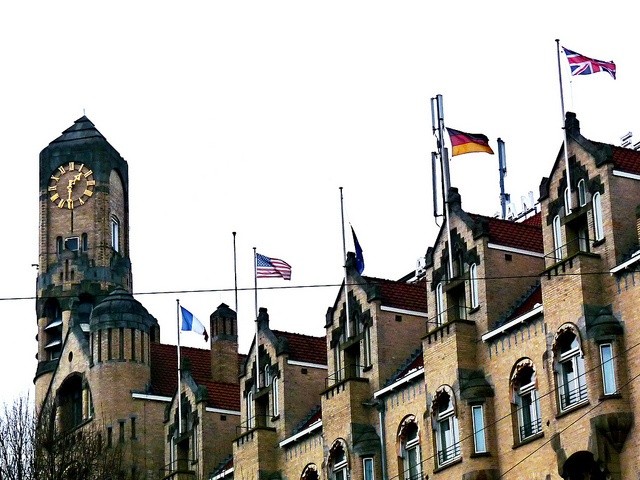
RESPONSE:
[47,162,95,209]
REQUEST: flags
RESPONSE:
[349,223,364,275]
[255,253,293,281]
[560,44,620,81]
[180,306,210,343]
[443,127,494,158]
